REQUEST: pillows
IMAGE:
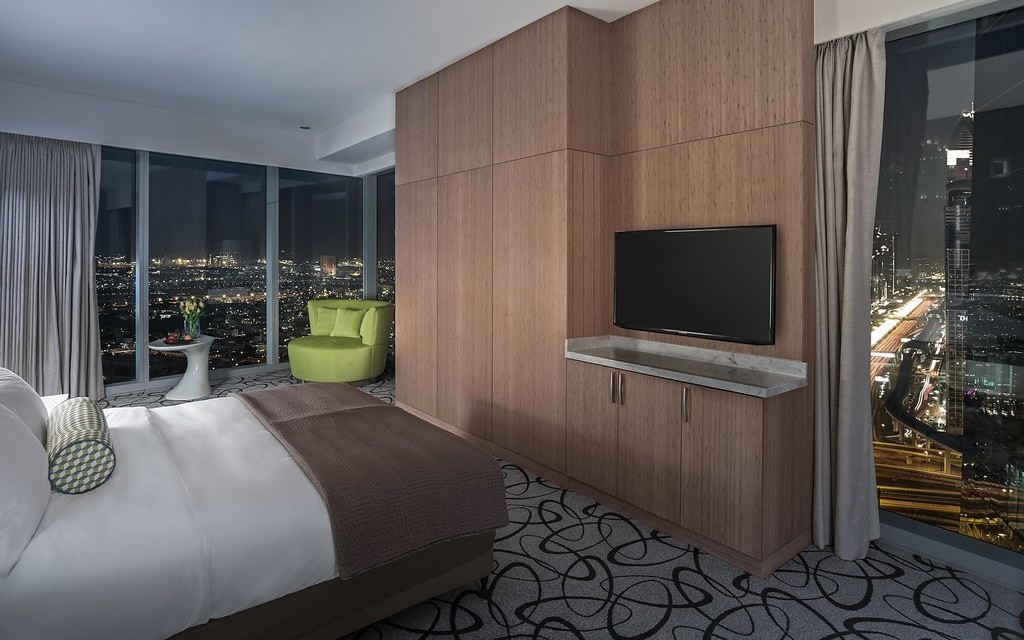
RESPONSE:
[43,396,116,495]
[314,306,339,336]
[0,367,49,447]
[0,404,51,581]
[330,307,366,339]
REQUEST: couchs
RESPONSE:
[286,297,394,384]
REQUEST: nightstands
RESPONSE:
[40,392,69,414]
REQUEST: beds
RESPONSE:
[0,382,509,640]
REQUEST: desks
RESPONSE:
[147,333,215,401]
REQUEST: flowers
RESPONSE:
[179,295,205,336]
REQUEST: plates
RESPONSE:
[163,340,194,345]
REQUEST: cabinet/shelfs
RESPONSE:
[396,5,817,579]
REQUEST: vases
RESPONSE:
[183,315,201,338]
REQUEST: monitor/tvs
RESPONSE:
[615,224,776,345]
